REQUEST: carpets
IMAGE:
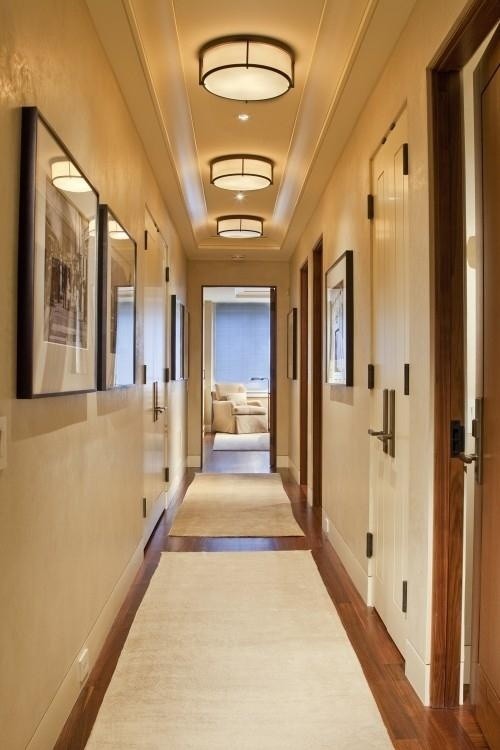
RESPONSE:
[212,432,270,451]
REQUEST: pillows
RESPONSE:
[226,392,247,406]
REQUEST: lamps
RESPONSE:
[197,32,297,239]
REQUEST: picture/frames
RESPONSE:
[323,249,355,387]
[16,105,138,399]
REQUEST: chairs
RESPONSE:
[210,383,268,434]
